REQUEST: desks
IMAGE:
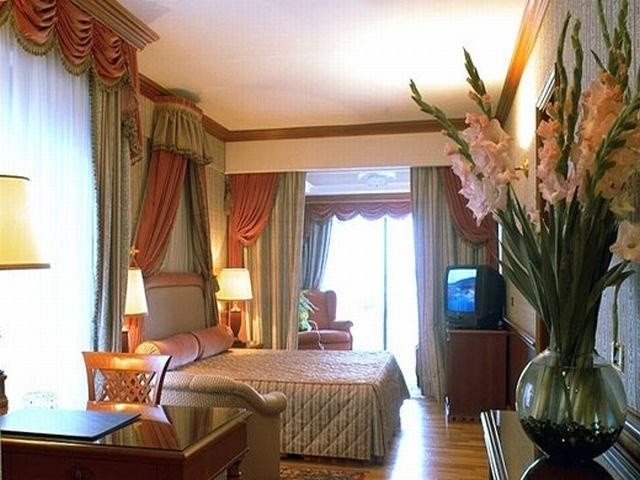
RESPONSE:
[442,329,510,425]
[481,409,640,480]
[0,403,251,480]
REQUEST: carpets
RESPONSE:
[280,464,370,480]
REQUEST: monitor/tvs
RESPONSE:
[443,265,507,328]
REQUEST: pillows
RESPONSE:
[135,333,199,370]
[192,324,235,360]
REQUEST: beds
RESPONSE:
[127,273,410,468]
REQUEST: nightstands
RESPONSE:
[247,341,265,348]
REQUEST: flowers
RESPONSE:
[409,0,640,429]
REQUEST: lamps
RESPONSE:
[214,268,253,348]
[122,266,148,352]
[0,175,51,415]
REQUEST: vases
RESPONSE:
[516,349,626,459]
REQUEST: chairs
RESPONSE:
[82,352,172,403]
[298,288,354,351]
[137,372,287,480]
